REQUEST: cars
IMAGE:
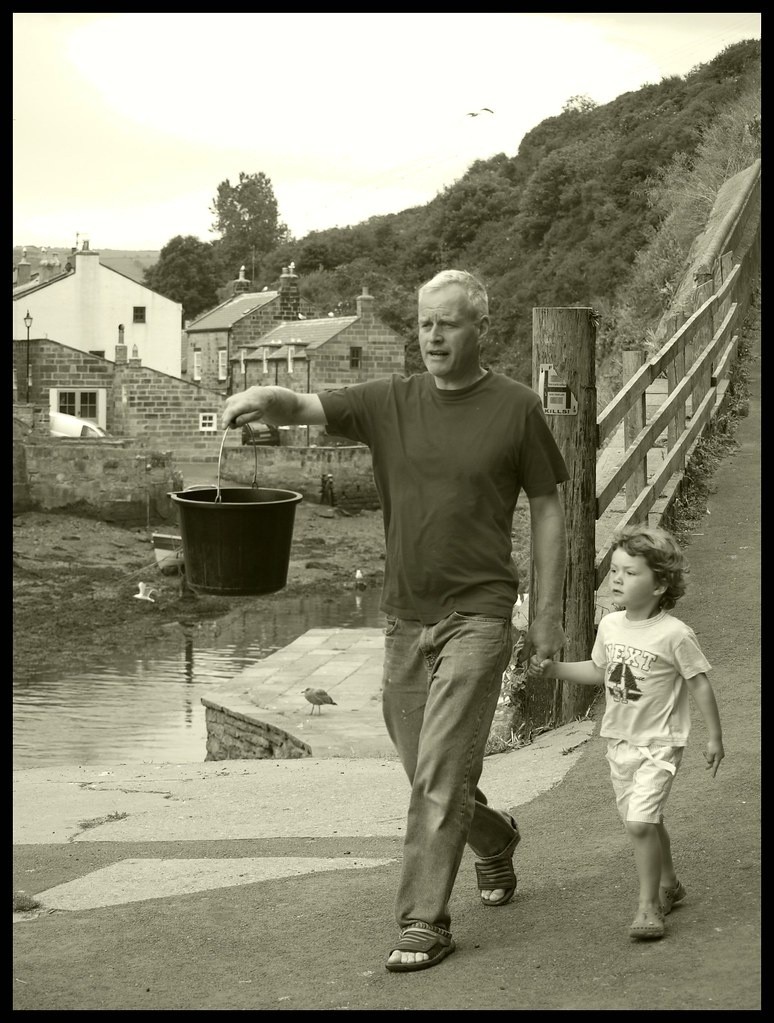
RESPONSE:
[240,421,281,445]
[47,411,111,440]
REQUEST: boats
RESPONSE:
[149,531,185,578]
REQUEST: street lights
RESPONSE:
[23,308,33,402]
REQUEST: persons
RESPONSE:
[527,523,727,942]
[218,269,569,971]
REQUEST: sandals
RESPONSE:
[474,856,518,905]
[385,930,458,970]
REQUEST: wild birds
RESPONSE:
[480,108,494,113]
[301,688,338,716]
[354,570,364,579]
[132,582,156,602]
[466,112,478,116]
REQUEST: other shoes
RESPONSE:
[661,880,686,914]
[627,904,665,937]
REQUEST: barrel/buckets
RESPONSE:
[165,419,302,597]
